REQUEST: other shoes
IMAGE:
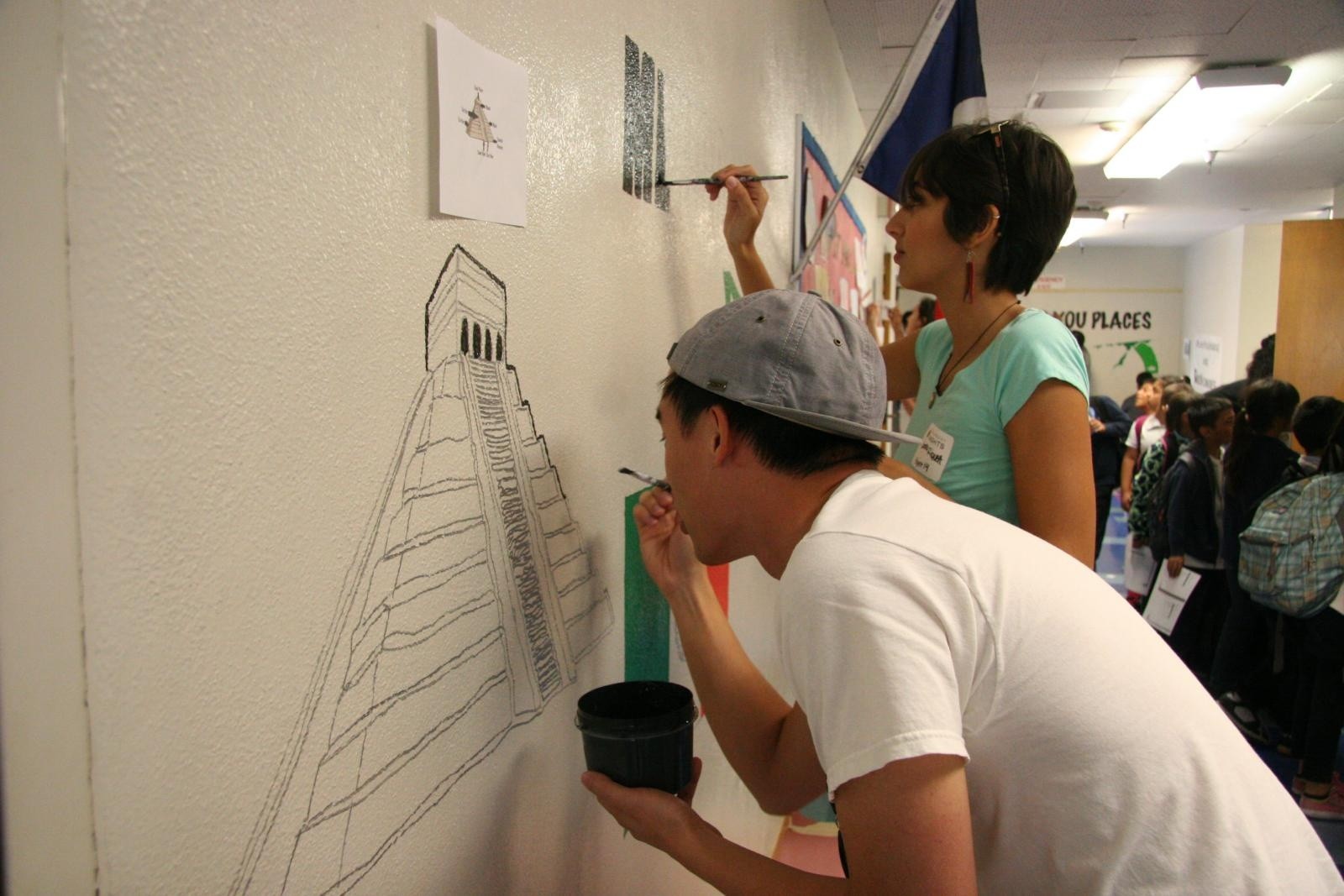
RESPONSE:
[1128,595,1143,614]
[1218,692,1265,742]
[1277,732,1296,753]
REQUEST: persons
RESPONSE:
[578,287,1343,895]
[865,295,1344,820]
[704,111,1097,577]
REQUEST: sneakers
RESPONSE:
[1300,771,1344,820]
[1292,775,1305,795]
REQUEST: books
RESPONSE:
[1124,531,1159,596]
[1142,558,1202,637]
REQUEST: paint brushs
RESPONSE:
[618,467,672,493]
[659,174,789,186]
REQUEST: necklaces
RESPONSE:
[927,299,1020,410]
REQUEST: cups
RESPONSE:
[574,679,699,798]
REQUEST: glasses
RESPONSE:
[977,120,1010,237]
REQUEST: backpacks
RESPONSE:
[1238,471,1344,619]
[1144,450,1209,561]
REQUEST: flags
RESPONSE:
[853,0,991,208]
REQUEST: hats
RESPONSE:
[668,291,922,445]
